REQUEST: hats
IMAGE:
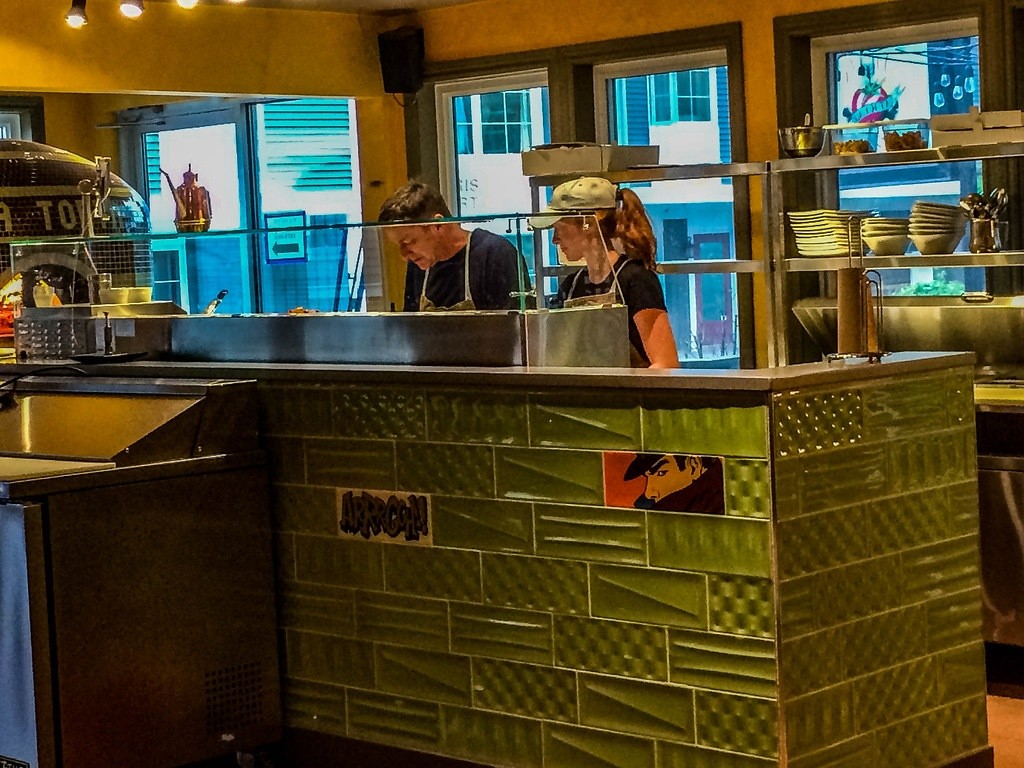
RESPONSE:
[527,176,619,229]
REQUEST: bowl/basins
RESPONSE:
[98,288,128,303]
[859,201,967,255]
[778,126,827,157]
[129,287,151,303]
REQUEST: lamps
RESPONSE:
[63,0,89,29]
[119,0,145,18]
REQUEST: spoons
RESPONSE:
[960,187,1008,219]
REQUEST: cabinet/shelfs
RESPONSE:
[528,143,1024,648]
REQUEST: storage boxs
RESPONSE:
[522,145,659,176]
[931,109,1024,145]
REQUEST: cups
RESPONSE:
[968,219,1002,254]
[87,273,111,305]
[33,286,54,307]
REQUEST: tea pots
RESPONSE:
[159,163,213,233]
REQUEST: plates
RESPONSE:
[787,209,873,256]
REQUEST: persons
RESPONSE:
[379,183,535,313]
[528,177,681,370]
